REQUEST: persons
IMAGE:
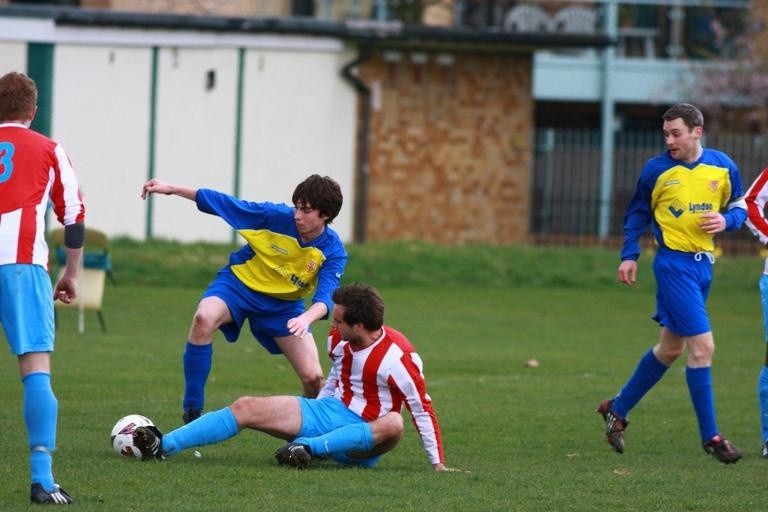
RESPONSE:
[140,169,347,433]
[132,281,468,475]
[0,70,86,506]
[744,165,768,463]
[596,97,749,469]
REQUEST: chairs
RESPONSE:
[53,229,115,334]
[552,7,598,36]
[503,4,552,33]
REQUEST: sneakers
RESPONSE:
[761,439,768,458]
[596,397,631,455]
[133,426,168,461]
[273,442,313,469]
[701,433,744,465]
[29,482,74,505]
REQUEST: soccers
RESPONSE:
[111,415,154,459]
